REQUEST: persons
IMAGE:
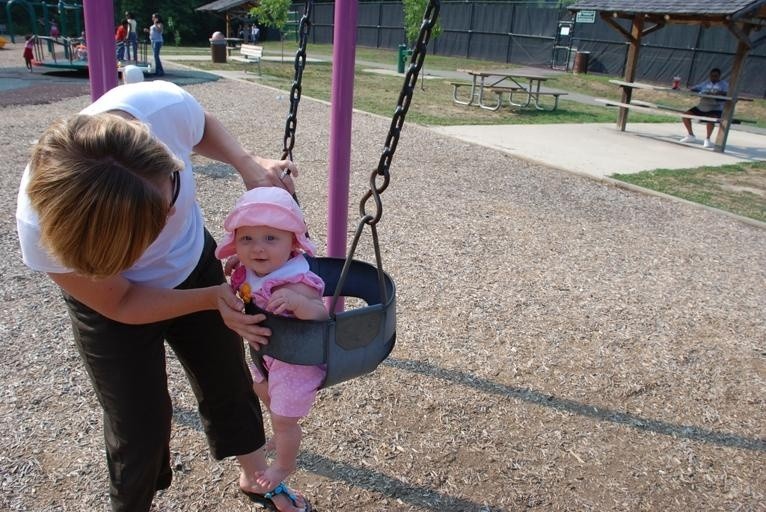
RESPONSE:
[143,13,165,76]
[681,68,730,148]
[12,80,319,512]
[115,20,130,61]
[213,185,330,490]
[48,18,60,38]
[230,23,260,47]
[21,34,37,71]
[124,11,139,62]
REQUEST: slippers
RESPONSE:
[239,480,312,512]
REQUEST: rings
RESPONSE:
[277,168,291,178]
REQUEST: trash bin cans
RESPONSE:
[574,52,592,75]
[209,32,227,63]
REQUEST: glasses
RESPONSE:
[170,169,180,208]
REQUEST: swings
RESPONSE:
[245,0,442,390]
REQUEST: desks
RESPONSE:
[456,68,557,106]
[609,79,754,103]
[208,38,245,56]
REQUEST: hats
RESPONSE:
[214,187,316,260]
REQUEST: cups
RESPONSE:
[672,77,681,89]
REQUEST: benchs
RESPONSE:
[594,98,724,123]
[226,46,241,50]
[443,80,526,106]
[630,99,758,125]
[479,86,568,112]
[227,43,263,75]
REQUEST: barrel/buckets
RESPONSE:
[210,44,227,62]
[573,50,591,73]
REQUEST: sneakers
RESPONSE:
[680,135,695,142]
[704,138,711,147]
